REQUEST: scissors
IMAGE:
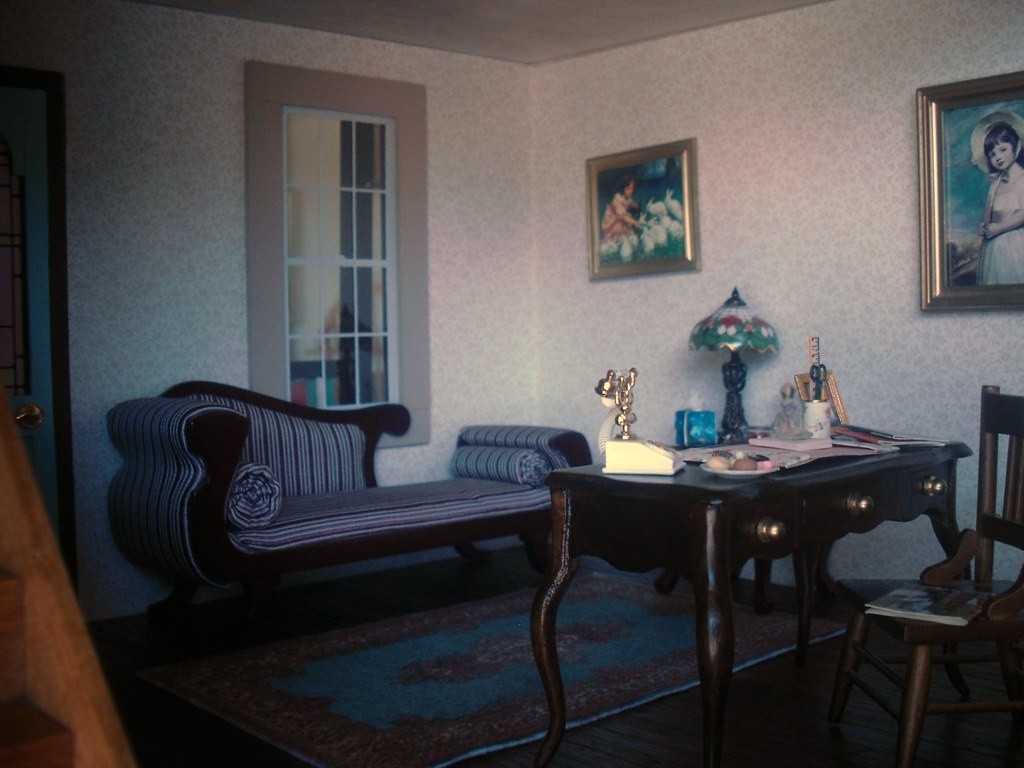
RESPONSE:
[809,364,827,400]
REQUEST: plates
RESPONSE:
[699,464,780,479]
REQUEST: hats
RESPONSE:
[970,110,1024,175]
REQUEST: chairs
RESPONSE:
[830,386,1024,768]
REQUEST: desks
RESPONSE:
[530,441,972,768]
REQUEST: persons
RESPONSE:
[601,175,641,243]
[970,108,1024,285]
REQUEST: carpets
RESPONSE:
[139,572,848,768]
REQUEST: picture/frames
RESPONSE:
[916,70,1024,311]
[585,137,701,281]
[794,370,848,426]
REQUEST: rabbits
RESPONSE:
[600,188,683,260]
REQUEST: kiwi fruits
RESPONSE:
[733,458,757,470]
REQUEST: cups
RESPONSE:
[802,398,831,439]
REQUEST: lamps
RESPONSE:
[689,287,778,444]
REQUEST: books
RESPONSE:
[864,584,996,627]
[749,424,949,451]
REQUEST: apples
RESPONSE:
[747,432,769,438]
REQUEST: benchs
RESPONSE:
[107,380,592,633]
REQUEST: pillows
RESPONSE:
[450,445,551,488]
[226,462,283,531]
